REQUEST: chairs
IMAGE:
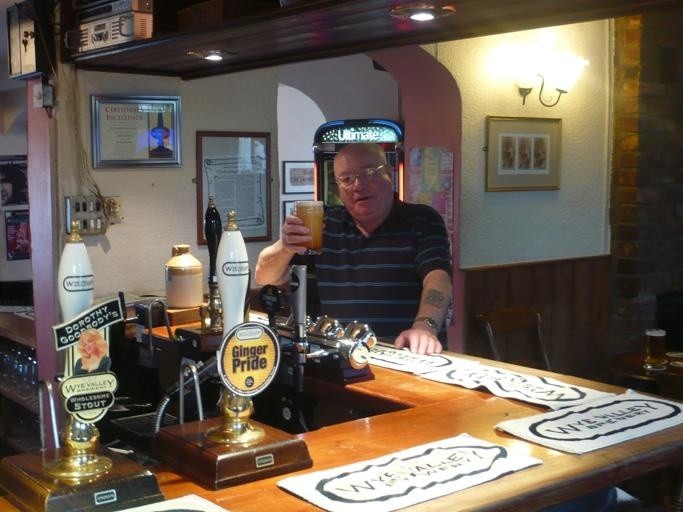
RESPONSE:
[480,304,552,371]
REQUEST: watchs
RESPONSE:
[415,314,441,332]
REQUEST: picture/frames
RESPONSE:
[192,131,274,246]
[486,115,563,192]
[5,209,30,261]
[283,161,315,194]
[91,94,181,169]
[283,200,316,223]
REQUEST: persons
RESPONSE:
[255,142,455,356]
[0,165,27,207]
[73,327,112,438]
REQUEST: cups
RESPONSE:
[644,330,667,372]
[296,202,324,255]
[0,348,37,395]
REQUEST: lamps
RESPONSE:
[482,30,589,106]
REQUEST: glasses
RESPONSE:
[336,164,386,186]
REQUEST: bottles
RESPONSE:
[164,244,205,309]
